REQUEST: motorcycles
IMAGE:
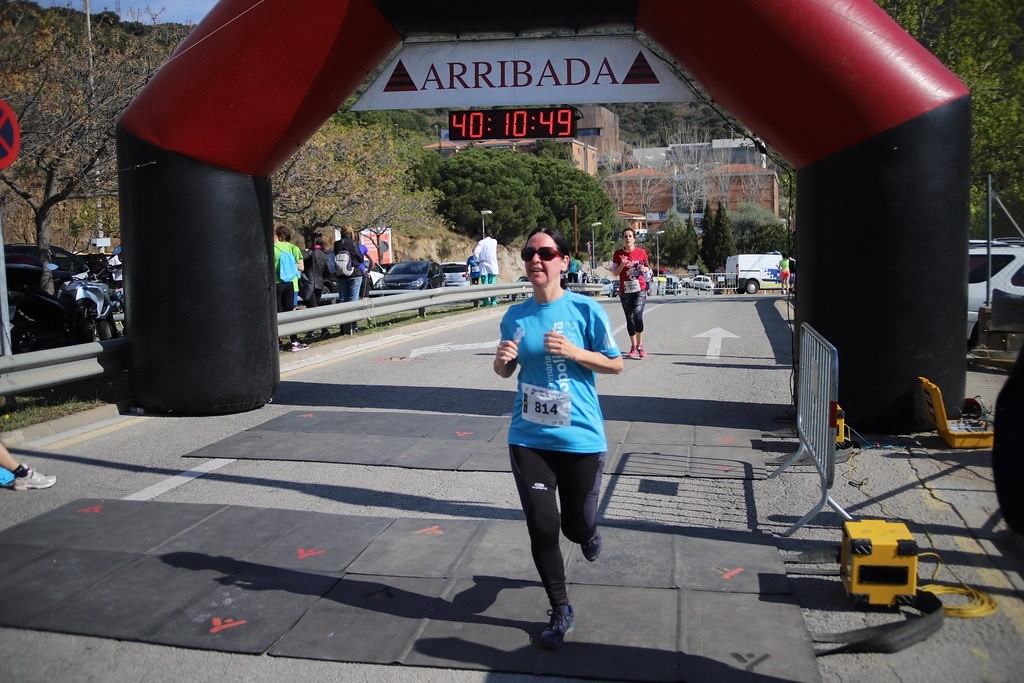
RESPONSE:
[10,246,124,354]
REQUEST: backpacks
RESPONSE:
[334,239,361,276]
[274,245,301,282]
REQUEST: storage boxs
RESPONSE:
[918,375,994,448]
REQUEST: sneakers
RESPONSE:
[539,604,575,649]
[636,344,648,357]
[14,467,58,491]
[580,530,605,561]
[290,342,310,352]
[628,346,638,358]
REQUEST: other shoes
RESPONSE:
[304,333,317,340]
[480,302,490,307]
[338,326,359,335]
[321,328,330,337]
[491,302,497,306]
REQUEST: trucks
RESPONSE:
[725,251,796,294]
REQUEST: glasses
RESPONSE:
[307,239,312,242]
[521,244,563,262]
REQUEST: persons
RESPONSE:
[353,232,375,328]
[0,441,56,491]
[274,226,309,351]
[776,252,790,295]
[644,268,653,296]
[334,225,364,334]
[564,252,587,283]
[473,228,499,307]
[493,227,624,649]
[612,227,651,358]
[302,232,330,338]
[465,249,482,301]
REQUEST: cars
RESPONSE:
[364,259,422,299]
[591,279,614,297]
[374,260,446,291]
[967,238,1024,351]
[612,280,620,297]
[507,276,529,298]
[5,244,86,294]
[681,275,715,291]
[652,268,682,294]
[440,262,471,287]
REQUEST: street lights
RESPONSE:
[657,230,665,280]
[481,211,492,239]
[592,222,602,278]
